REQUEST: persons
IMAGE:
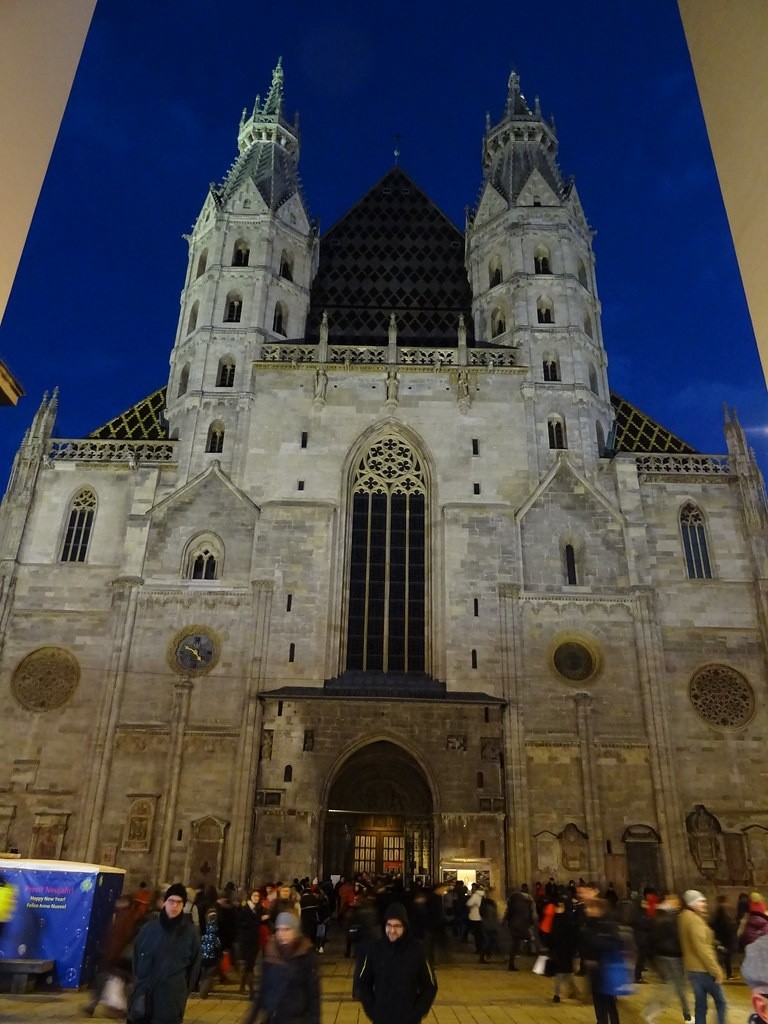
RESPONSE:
[1,865,768,1024]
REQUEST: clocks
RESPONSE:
[174,631,217,672]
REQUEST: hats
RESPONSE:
[162,882,187,908]
[273,912,300,931]
[739,934,768,995]
[384,902,409,928]
[682,890,707,906]
[749,892,765,914]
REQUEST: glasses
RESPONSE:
[164,899,184,906]
[384,922,404,929]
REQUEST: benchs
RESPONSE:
[0,955,56,995]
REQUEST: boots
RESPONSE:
[238,966,255,1001]
[198,975,212,998]
